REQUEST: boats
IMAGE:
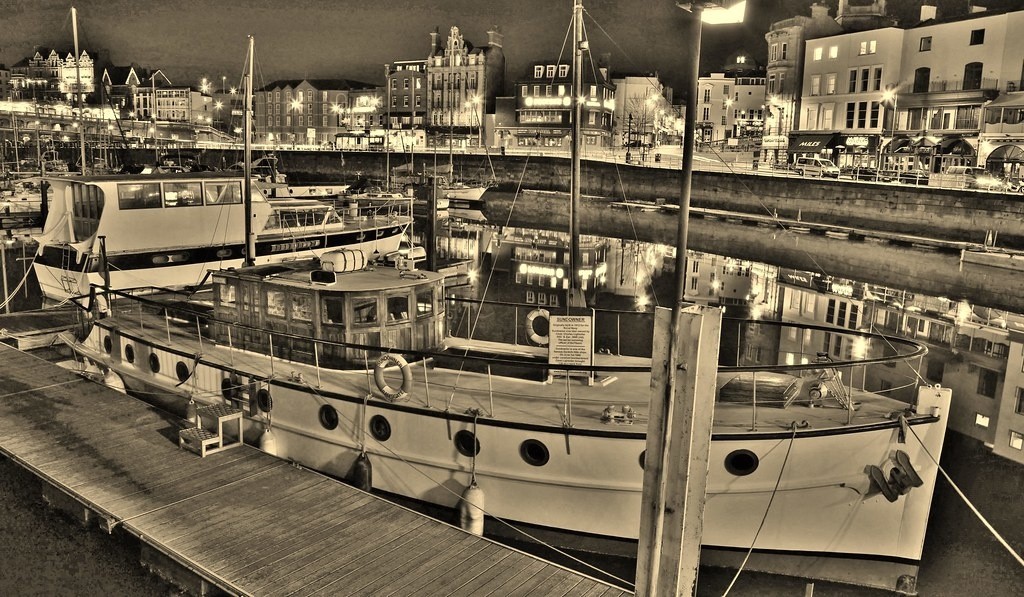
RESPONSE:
[1,9,499,309]
[59,0,956,597]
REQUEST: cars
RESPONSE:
[895,172,929,185]
[851,166,880,181]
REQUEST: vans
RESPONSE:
[947,165,990,189]
[794,156,839,178]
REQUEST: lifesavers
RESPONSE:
[374,353,412,400]
[524,309,549,344]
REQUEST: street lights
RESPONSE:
[466,96,480,139]
[883,81,899,154]
[645,0,749,597]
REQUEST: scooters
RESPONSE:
[1002,175,1024,193]
